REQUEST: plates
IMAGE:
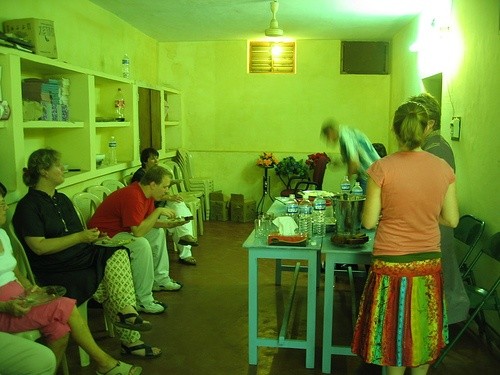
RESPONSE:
[95,117,114,122]
[94,239,130,247]
[26,286,66,306]
[165,218,185,223]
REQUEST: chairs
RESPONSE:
[429,215,500,373]
[281,157,330,198]
[7,150,215,375]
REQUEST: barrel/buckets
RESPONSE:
[328,194,366,236]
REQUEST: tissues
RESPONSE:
[267,216,310,248]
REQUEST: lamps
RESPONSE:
[421,71,442,129]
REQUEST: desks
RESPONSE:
[243,198,376,373]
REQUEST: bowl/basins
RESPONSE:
[298,190,334,203]
[96,154,105,162]
[326,223,337,232]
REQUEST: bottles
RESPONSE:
[351,182,364,200]
[108,136,117,164]
[326,200,333,217]
[285,194,299,234]
[312,195,326,237]
[299,195,312,238]
[340,175,351,200]
[114,88,125,121]
[121,54,129,77]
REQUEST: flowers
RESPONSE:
[258,152,279,166]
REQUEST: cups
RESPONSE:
[254,212,278,244]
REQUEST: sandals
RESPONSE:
[120,341,161,358]
[114,311,151,331]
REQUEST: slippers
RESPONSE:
[96,361,142,375]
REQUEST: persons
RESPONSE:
[322,123,382,192]
[0,332,56,375]
[411,92,470,324]
[0,183,142,375]
[352,102,460,375]
[13,149,162,358]
[86,165,190,313]
[140,148,199,265]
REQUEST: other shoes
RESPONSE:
[136,299,168,314]
[179,256,196,265]
[178,235,198,246]
[153,279,182,291]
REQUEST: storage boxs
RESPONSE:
[3,18,57,59]
[230,194,256,222]
[209,191,231,222]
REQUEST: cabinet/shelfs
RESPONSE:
[0,46,185,278]
